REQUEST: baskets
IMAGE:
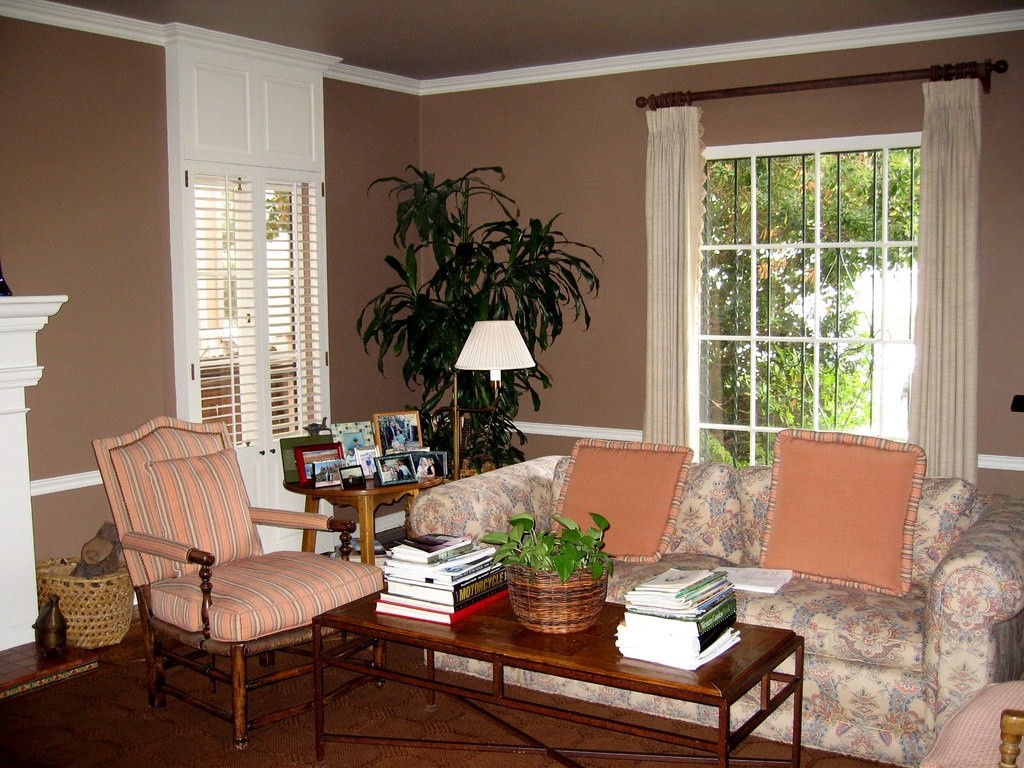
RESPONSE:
[35,557,133,648]
[508,558,608,634]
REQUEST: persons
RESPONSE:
[326,472,333,483]
[417,454,444,477]
[388,420,406,449]
[382,458,413,481]
[366,454,376,475]
[347,437,363,464]
[348,474,356,484]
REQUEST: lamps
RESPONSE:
[453,321,538,467]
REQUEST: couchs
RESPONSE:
[412,451,1024,768]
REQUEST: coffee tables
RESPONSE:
[312,590,804,768]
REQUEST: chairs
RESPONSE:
[92,415,383,750]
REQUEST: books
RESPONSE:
[330,537,387,567]
[373,533,509,626]
[713,565,793,593]
[614,568,742,671]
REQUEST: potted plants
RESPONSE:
[481,512,613,633]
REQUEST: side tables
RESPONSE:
[282,476,446,566]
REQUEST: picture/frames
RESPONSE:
[373,452,418,485]
[294,443,345,487]
[331,421,375,465]
[374,409,424,456]
[355,445,380,478]
[338,465,367,490]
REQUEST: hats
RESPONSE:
[353,438,358,441]
[348,446,353,450]
